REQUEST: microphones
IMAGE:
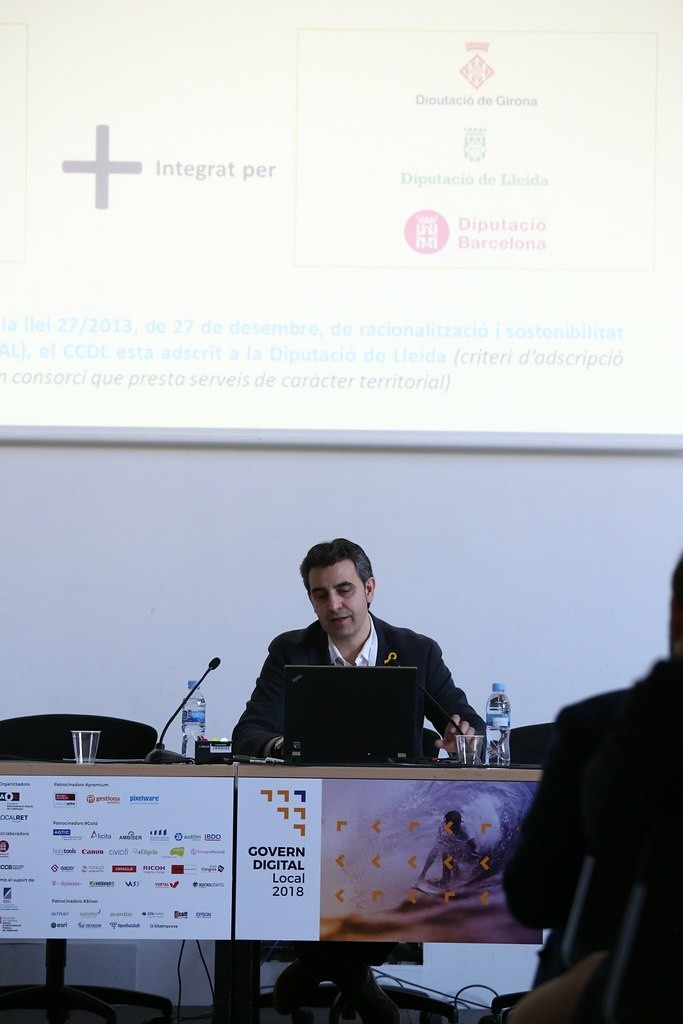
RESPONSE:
[389,659,482,766]
[144,658,221,763]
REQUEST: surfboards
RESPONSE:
[410,867,483,894]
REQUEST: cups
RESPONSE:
[455,735,485,765]
[72,730,101,765]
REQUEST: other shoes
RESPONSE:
[272,956,331,1016]
[335,965,402,1024]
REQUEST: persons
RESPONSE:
[417,810,478,888]
[231,538,490,1024]
[504,547,683,1022]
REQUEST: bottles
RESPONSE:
[484,681,511,767]
[180,680,207,763]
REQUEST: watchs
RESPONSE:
[273,736,285,760]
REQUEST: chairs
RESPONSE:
[0,715,177,1024]
[257,727,445,1022]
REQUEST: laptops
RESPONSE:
[283,664,418,767]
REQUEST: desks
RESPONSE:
[0,753,545,946]
